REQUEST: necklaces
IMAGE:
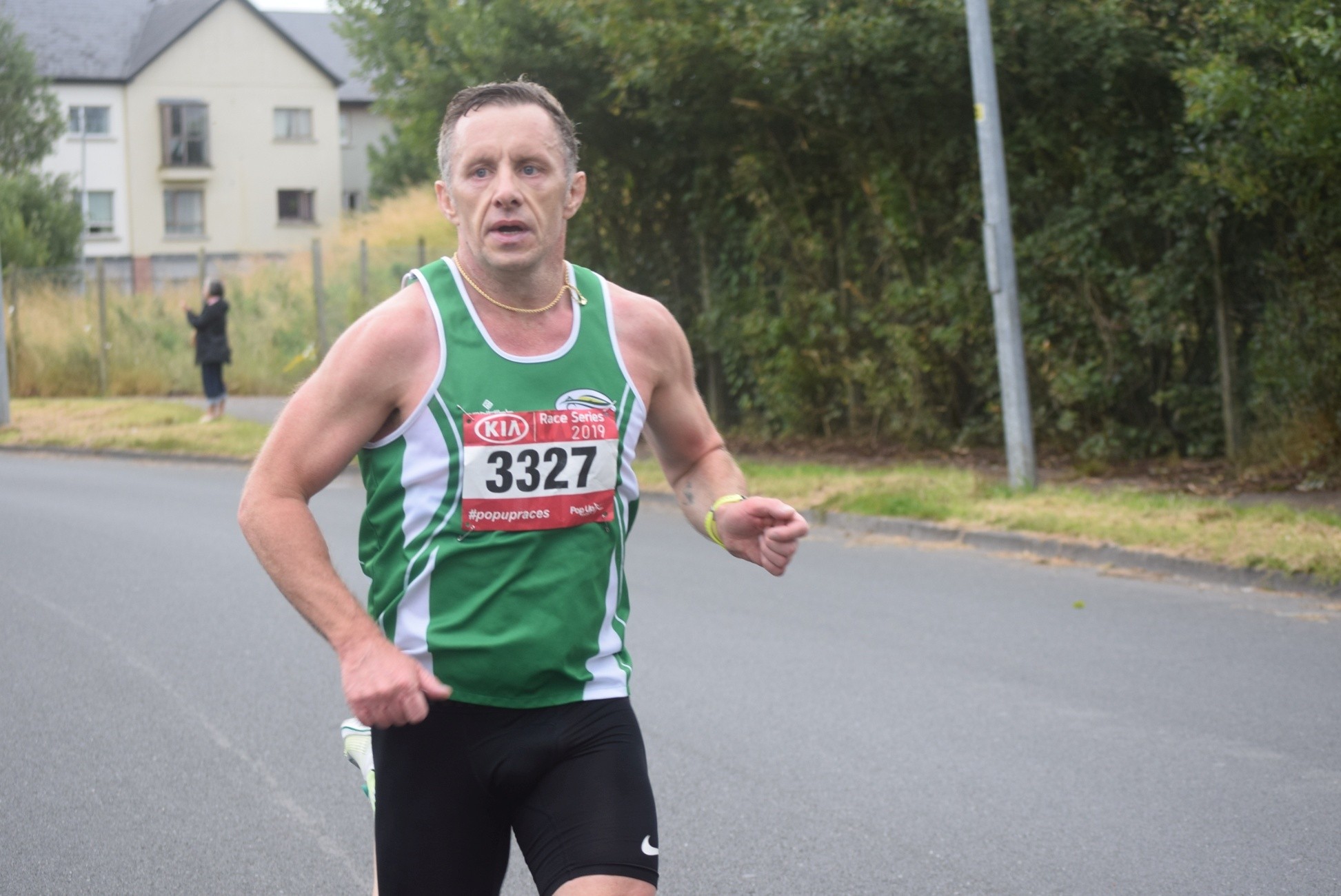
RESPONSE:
[454,251,588,314]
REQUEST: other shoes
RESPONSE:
[338,716,376,810]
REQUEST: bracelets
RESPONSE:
[704,493,748,549]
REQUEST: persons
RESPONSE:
[237,82,812,895]
[182,279,232,424]
